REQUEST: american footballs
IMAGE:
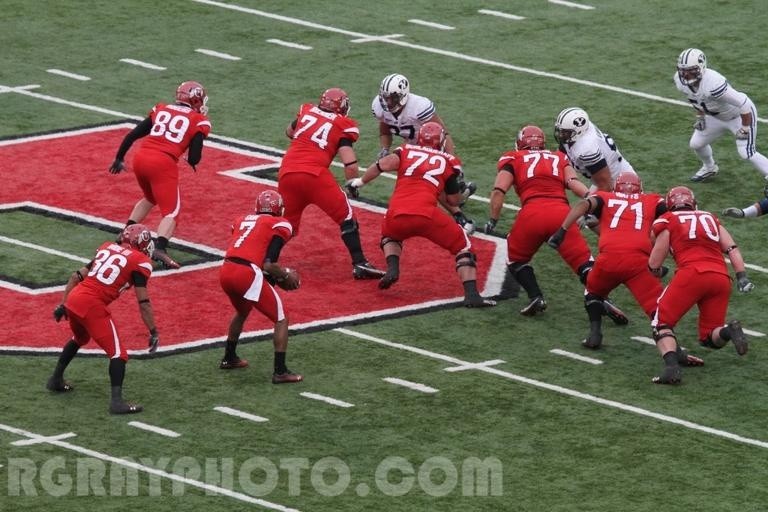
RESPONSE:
[272,267,301,290]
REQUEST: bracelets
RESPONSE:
[741,205,758,218]
[735,271,747,280]
[150,326,159,335]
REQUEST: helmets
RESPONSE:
[614,172,642,194]
[418,122,447,151]
[666,185,696,212]
[676,48,706,85]
[119,224,155,259]
[255,190,284,217]
[379,73,410,113]
[319,88,350,116]
[515,125,545,151]
[175,81,208,117]
[555,107,589,143]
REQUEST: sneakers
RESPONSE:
[220,358,248,368]
[582,335,601,348]
[110,403,142,413]
[378,271,399,289]
[465,297,497,308]
[519,295,547,316]
[353,261,386,279]
[150,248,180,269]
[272,372,303,383]
[603,300,628,325]
[678,350,704,365]
[47,378,74,392]
[729,319,748,355]
[463,218,477,233]
[652,368,681,384]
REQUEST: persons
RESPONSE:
[721,183,768,219]
[546,171,704,367]
[219,190,304,383]
[555,107,669,277]
[344,122,497,308]
[109,81,212,268]
[276,87,387,279]
[46,223,160,414]
[673,47,768,184]
[485,125,629,327]
[371,73,477,236]
[651,187,754,384]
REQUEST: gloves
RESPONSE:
[722,206,745,219]
[54,304,68,322]
[650,267,669,278]
[735,125,750,139]
[148,331,158,353]
[735,270,755,293]
[546,228,567,249]
[462,182,477,196]
[378,148,389,158]
[345,176,364,199]
[576,214,600,230]
[109,160,127,175]
[485,217,497,234]
[693,114,705,130]
